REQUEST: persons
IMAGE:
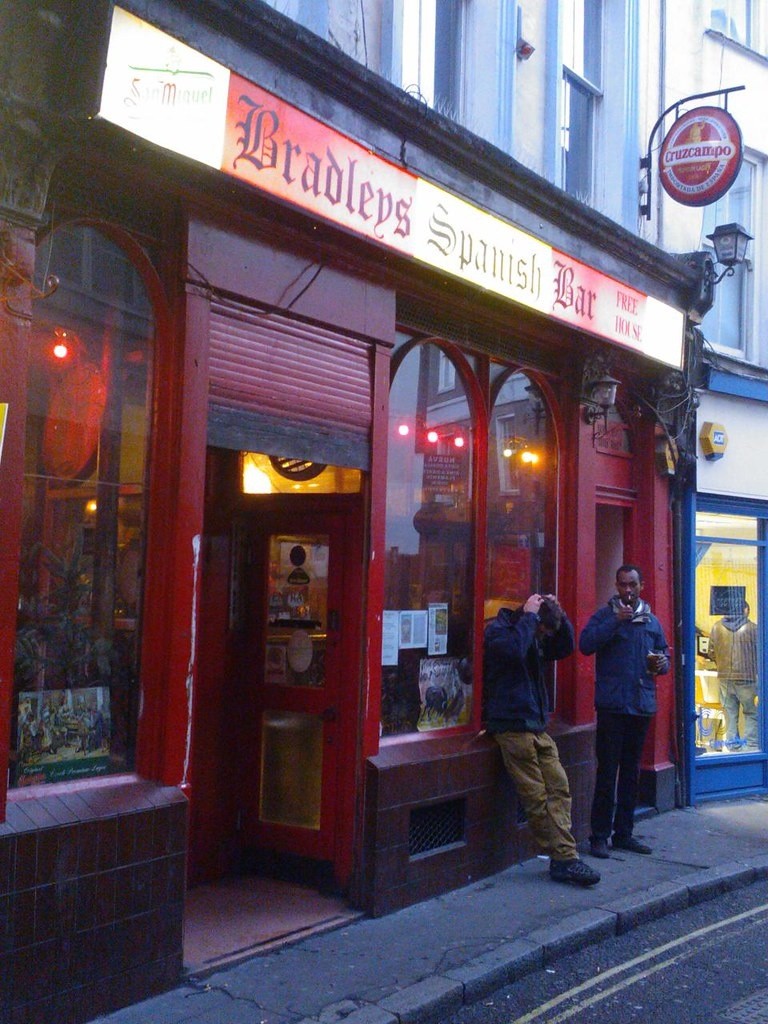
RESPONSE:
[483,592,599,886]
[707,599,762,754]
[577,562,671,858]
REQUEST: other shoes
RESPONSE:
[590,844,610,858]
[613,838,652,855]
[560,859,600,885]
[549,860,560,881]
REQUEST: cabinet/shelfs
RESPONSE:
[31,482,144,691]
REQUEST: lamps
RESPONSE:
[525,384,545,434]
[584,368,622,432]
[705,222,754,285]
